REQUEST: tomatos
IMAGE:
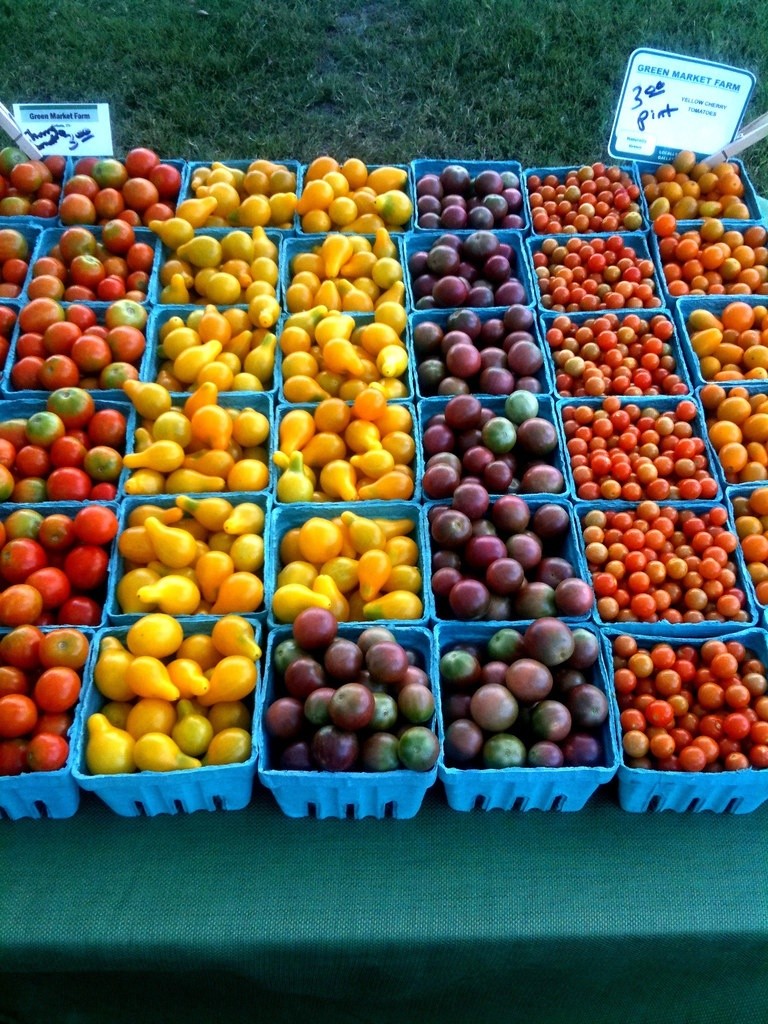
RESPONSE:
[0,147,768,777]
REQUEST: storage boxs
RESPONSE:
[0,157,768,817]
